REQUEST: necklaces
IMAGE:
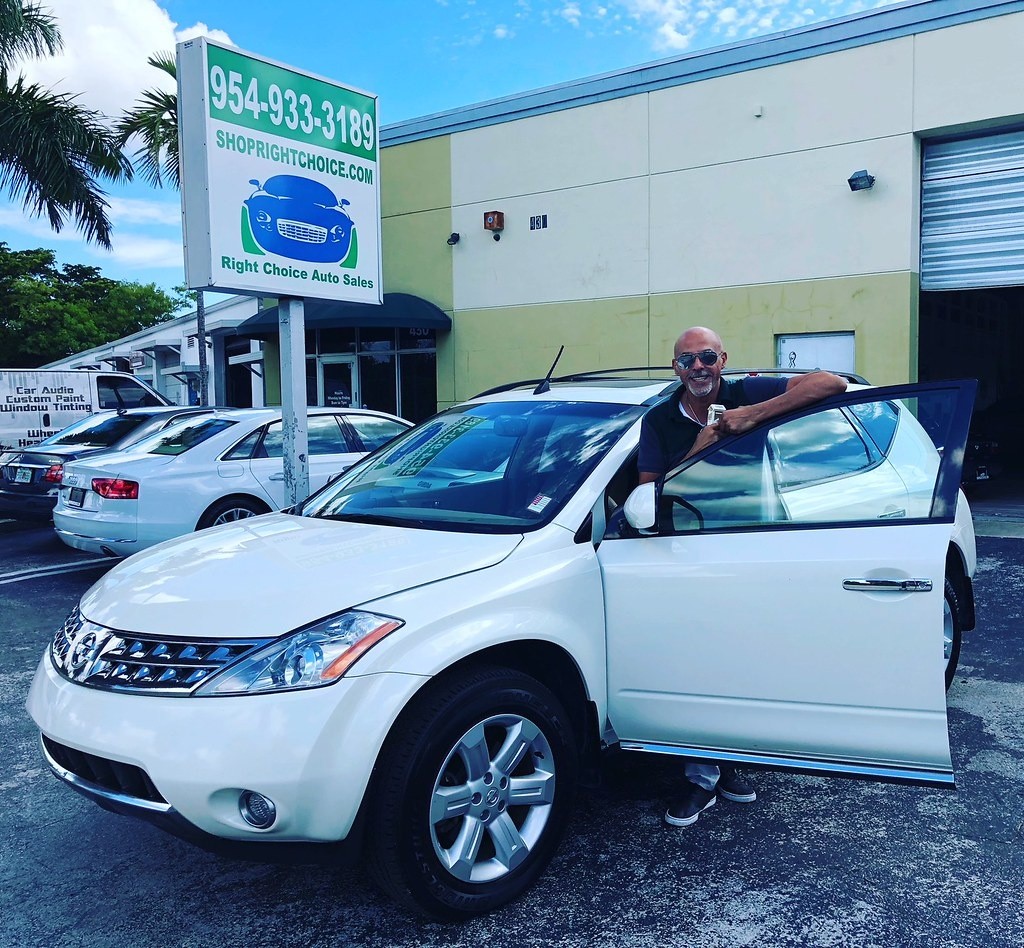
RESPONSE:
[686,394,701,423]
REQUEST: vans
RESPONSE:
[0,369,177,450]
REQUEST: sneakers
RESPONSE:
[719,771,756,803]
[664,785,717,826]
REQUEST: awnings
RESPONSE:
[236,292,452,338]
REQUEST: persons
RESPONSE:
[638,327,847,826]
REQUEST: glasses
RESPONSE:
[676,350,723,371]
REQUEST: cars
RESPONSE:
[0,408,238,524]
[25,356,982,920]
[384,416,600,504]
[51,404,415,558]
